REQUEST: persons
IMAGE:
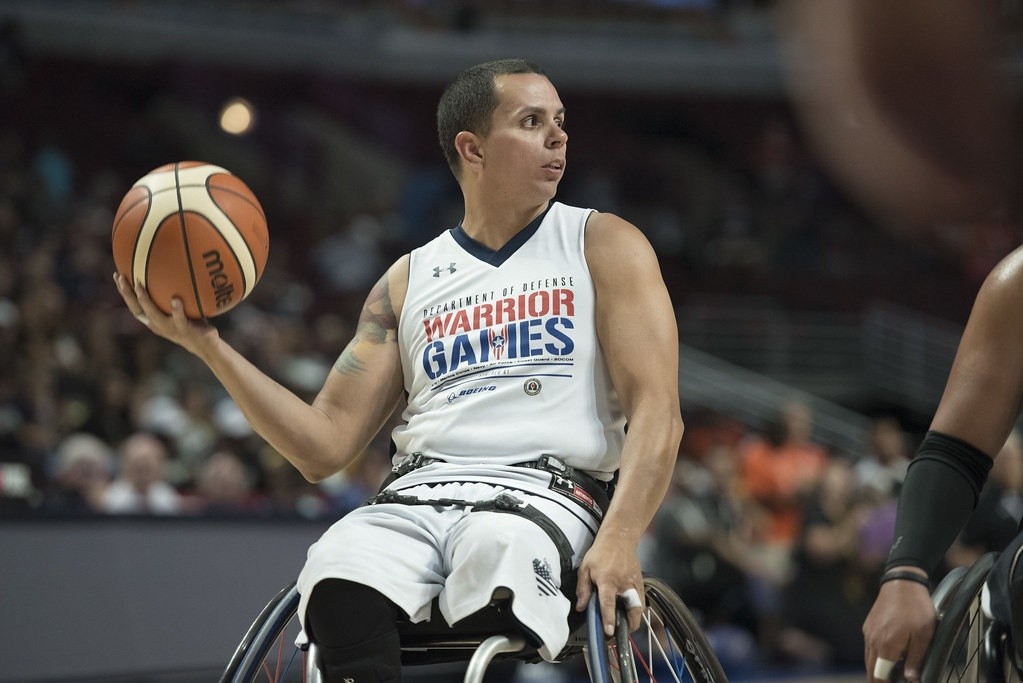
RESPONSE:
[0,207,408,518]
[862,247,1022,683]
[111,61,684,683]
[639,405,1023,666]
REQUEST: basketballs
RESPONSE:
[110,158,272,322]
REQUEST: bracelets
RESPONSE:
[880,571,930,588]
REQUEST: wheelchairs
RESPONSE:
[885,532,1022,683]
[219,566,730,683]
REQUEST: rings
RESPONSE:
[873,657,895,679]
[135,314,150,326]
[622,588,642,609]
